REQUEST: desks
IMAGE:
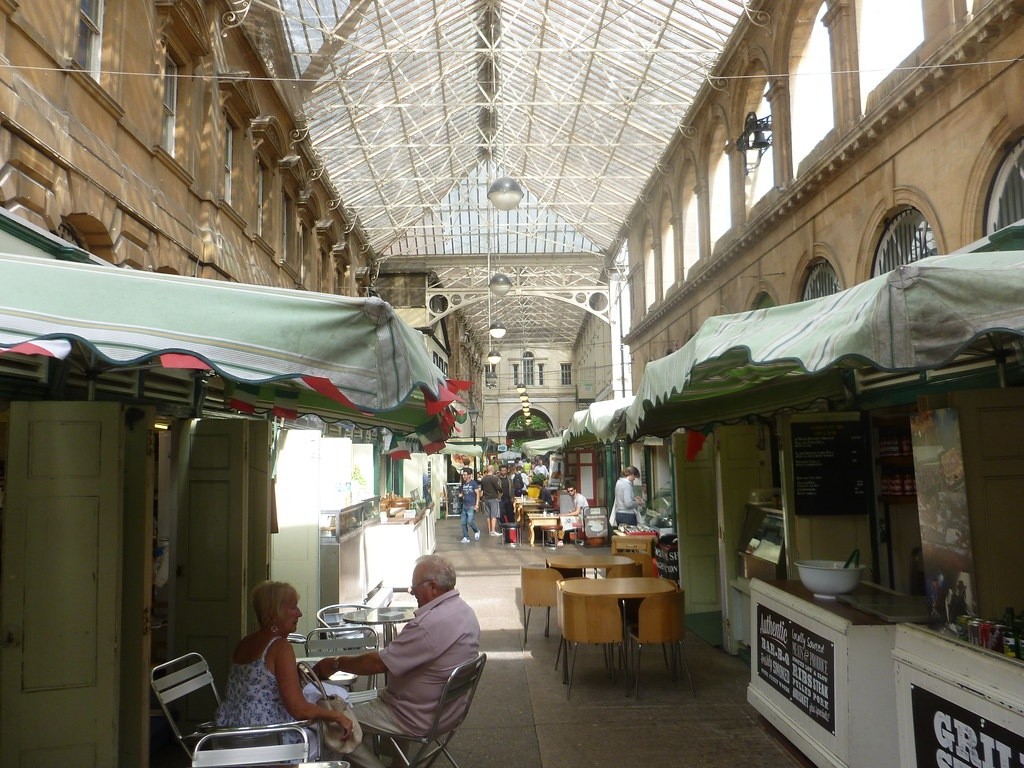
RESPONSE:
[560,577,676,688]
[324,669,356,694]
[611,533,653,556]
[526,512,560,546]
[342,606,418,686]
[513,495,547,529]
[547,555,637,651]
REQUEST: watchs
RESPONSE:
[332,657,338,673]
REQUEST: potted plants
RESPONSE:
[531,472,546,487]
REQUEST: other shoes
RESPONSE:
[474,529,480,540]
[489,531,502,537]
[460,537,471,543]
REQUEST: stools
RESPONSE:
[542,525,564,548]
[501,523,522,547]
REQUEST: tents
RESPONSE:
[498,451,521,464]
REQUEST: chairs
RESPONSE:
[305,625,381,758]
[614,553,653,578]
[150,652,223,758]
[519,564,564,651]
[191,725,309,768]
[605,562,644,579]
[198,721,323,768]
[628,589,697,700]
[560,590,628,698]
[316,603,398,691]
[360,653,487,768]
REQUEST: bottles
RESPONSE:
[1002,607,1024,661]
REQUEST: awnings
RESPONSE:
[439,443,482,458]
[522,250,1024,458]
[0,252,474,460]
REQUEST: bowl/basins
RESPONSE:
[794,560,866,600]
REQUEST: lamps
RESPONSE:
[487,337,502,365]
[516,300,526,393]
[522,406,529,412]
[522,400,530,407]
[488,0,524,212]
[488,205,513,297]
[523,411,530,416]
[525,416,532,426]
[485,369,498,388]
[489,294,506,339]
[520,393,529,401]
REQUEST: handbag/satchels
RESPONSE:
[609,496,618,526]
[297,661,363,753]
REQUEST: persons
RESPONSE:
[615,466,643,553]
[211,580,353,768]
[306,554,481,768]
[458,457,589,547]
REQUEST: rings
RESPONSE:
[352,729,354,732]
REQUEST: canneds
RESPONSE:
[958,615,1007,652]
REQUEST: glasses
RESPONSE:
[567,489,574,492]
[411,579,432,591]
